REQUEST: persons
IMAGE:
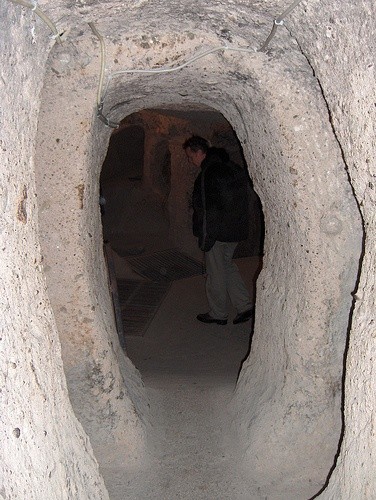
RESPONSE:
[182,136,256,325]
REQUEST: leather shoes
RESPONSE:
[198,308,255,325]
